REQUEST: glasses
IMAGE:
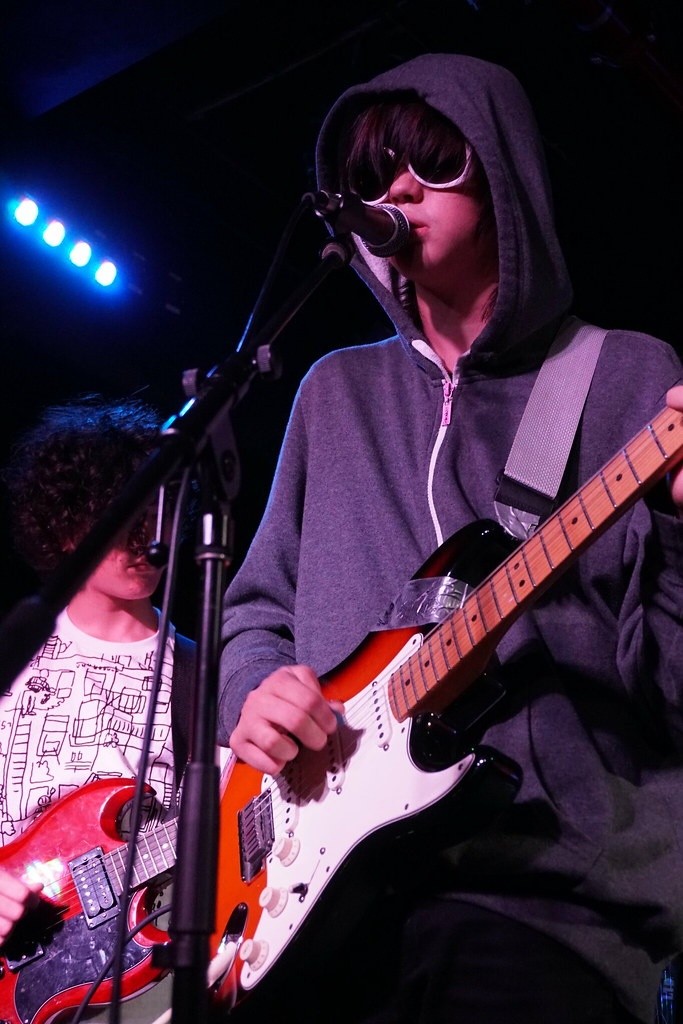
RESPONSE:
[340,130,491,206]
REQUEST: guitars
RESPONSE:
[203,393,683,1009]
[0,774,183,1024]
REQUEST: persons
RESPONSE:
[0,404,200,1024]
[217,52,683,1024]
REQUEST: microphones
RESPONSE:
[302,189,411,258]
